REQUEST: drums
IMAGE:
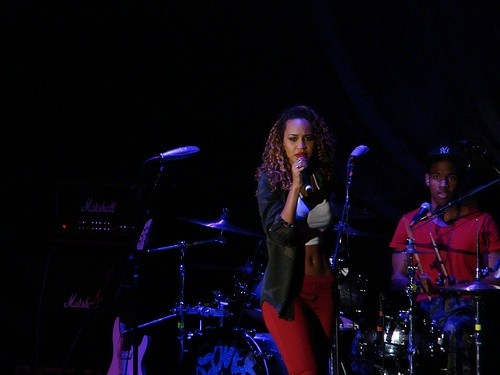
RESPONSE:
[176,302,233,361]
[333,266,368,303]
[183,325,288,375]
[209,267,263,319]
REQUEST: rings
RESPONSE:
[296,165,299,168]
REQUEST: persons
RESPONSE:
[255,105,342,375]
[390,149,500,346]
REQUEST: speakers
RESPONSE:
[33,240,134,370]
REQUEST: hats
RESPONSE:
[427,146,460,163]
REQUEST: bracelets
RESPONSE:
[277,215,295,228]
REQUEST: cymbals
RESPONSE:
[179,214,267,239]
[437,282,492,291]
[328,225,378,238]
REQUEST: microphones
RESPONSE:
[302,173,313,194]
[350,145,370,160]
[148,146,200,164]
[410,202,431,228]
[376,312,384,345]
